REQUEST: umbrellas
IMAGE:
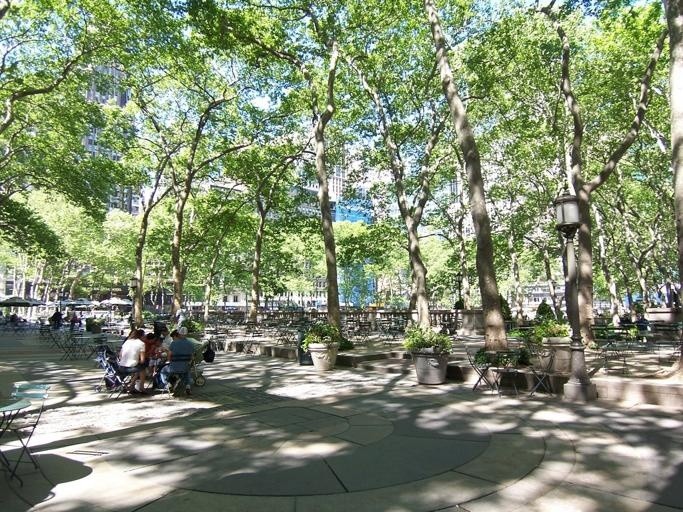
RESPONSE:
[0,295,131,309]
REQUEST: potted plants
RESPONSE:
[300,318,354,371]
[633,302,648,330]
[531,318,573,372]
[499,294,513,330]
[403,320,453,385]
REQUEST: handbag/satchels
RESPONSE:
[203,341,215,362]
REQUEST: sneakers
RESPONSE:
[166,382,173,393]
[184,389,192,395]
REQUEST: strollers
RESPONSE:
[191,337,215,386]
[94,345,120,392]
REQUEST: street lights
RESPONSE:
[552,181,590,385]
[129,276,138,320]
[455,271,464,309]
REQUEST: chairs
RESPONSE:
[465,345,496,393]
[590,322,683,365]
[0,318,197,487]
[203,318,301,347]
[342,316,407,348]
[527,347,556,399]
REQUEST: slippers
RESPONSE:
[129,390,148,394]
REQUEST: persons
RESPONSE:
[5,308,85,331]
[119,324,192,397]
[634,313,651,344]
[611,311,621,333]
[175,304,190,325]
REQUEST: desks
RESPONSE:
[495,368,527,399]
